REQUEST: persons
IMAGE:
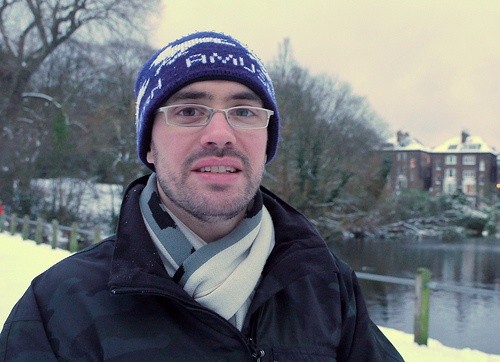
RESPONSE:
[0,31,405,362]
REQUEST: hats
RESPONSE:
[135,31,280,172]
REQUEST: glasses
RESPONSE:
[157,104,274,130]
